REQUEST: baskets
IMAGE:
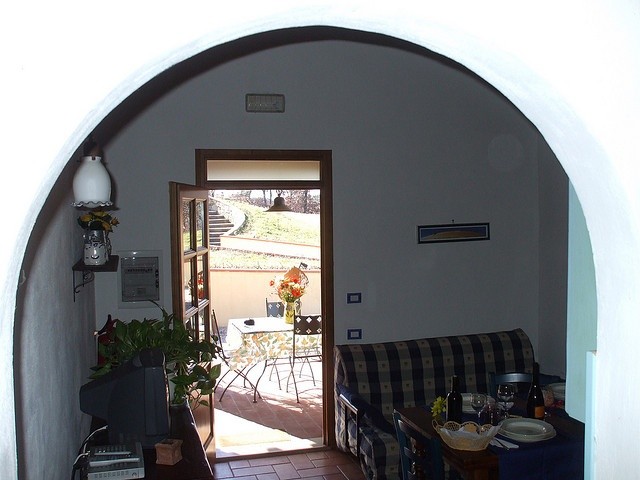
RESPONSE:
[431,419,504,452]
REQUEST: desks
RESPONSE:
[227,315,322,403]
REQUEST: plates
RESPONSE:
[547,382,566,398]
[497,424,557,443]
[501,418,554,438]
[460,393,495,414]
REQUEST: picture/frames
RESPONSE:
[418,223,490,244]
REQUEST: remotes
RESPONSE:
[89,455,140,467]
[94,445,134,455]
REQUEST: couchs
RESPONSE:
[332,327,565,480]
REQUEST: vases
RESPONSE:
[82,229,112,267]
[285,300,295,324]
[293,298,303,316]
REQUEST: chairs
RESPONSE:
[391,411,445,479]
[486,362,541,407]
[286,310,322,403]
[265,297,285,383]
[211,308,264,403]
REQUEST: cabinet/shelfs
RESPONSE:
[80,396,215,480]
[71,253,118,302]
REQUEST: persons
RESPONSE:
[220,192,224,199]
[210,190,215,198]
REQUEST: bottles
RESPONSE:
[446,376,463,425]
[523,363,545,422]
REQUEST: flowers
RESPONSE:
[187,270,205,299]
[77,210,120,232]
[283,265,309,298]
[269,276,307,302]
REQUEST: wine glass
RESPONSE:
[498,383,517,419]
[471,392,487,426]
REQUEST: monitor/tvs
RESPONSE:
[80,347,172,449]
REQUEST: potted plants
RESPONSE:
[88,298,222,413]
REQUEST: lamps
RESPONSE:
[246,93,285,113]
[69,134,114,209]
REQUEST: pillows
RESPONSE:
[336,383,385,430]
